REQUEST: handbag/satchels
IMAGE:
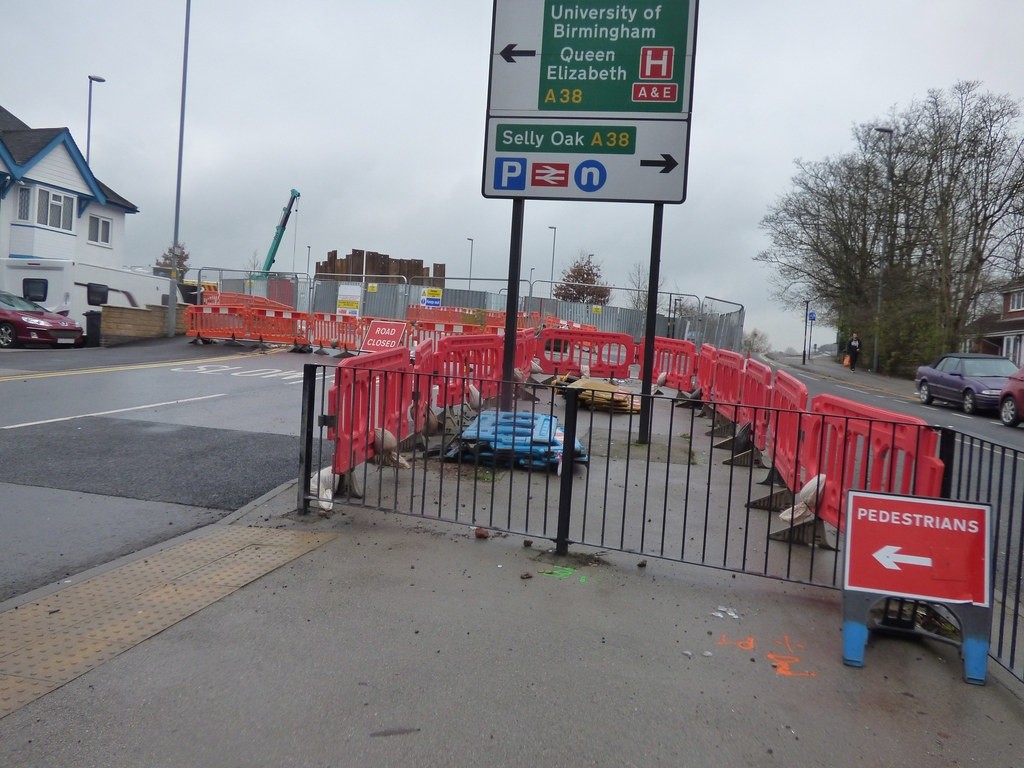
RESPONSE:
[844,355,850,366]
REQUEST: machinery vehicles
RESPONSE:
[247,189,300,278]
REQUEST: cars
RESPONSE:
[915,352,1021,414]
[0,290,84,349]
[998,365,1024,428]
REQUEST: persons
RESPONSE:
[847,332,861,371]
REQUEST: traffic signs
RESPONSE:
[481,1,699,206]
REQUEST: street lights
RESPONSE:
[548,226,556,299]
[307,246,311,276]
[672,298,682,338]
[588,254,594,265]
[801,300,811,364]
[84,75,107,166]
[466,237,474,290]
[529,268,535,283]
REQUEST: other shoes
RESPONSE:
[851,368,855,372]
